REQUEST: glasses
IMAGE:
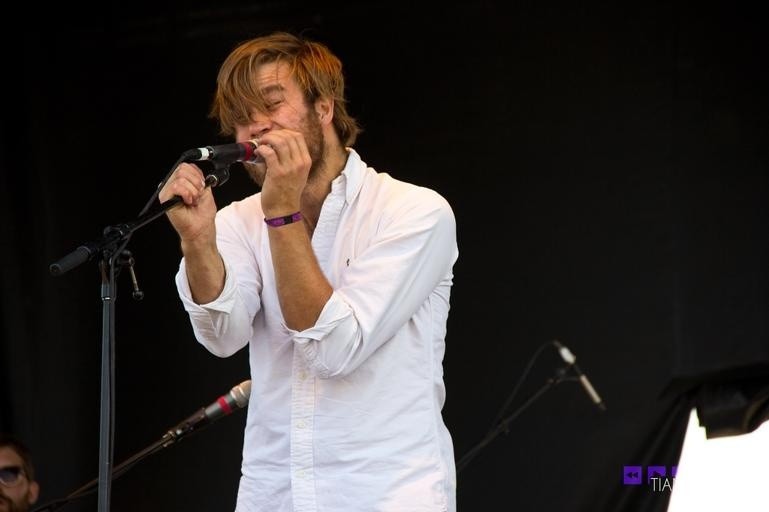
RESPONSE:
[0,465,30,487]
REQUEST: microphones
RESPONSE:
[553,338,608,414]
[163,380,252,443]
[185,139,273,164]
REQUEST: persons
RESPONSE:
[0,429,40,511]
[157,32,461,512]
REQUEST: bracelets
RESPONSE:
[264,210,304,227]
